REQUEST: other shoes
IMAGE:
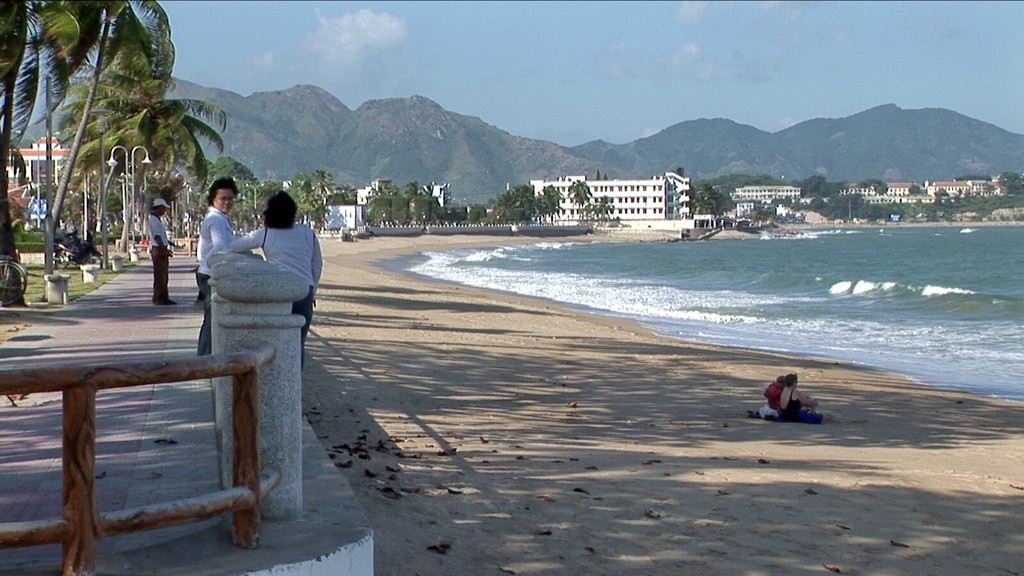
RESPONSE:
[195,300,203,304]
[152,300,178,305]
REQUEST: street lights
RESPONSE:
[105,145,153,255]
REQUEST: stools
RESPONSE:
[130,250,140,261]
[80,265,100,283]
[110,255,125,271]
[44,274,70,302]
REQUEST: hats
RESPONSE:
[774,375,785,388]
[152,198,170,208]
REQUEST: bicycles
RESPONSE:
[0,254,28,306]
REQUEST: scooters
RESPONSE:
[53,230,102,271]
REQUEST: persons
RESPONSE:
[195,177,244,356]
[229,189,322,370]
[149,198,178,306]
[763,374,833,425]
